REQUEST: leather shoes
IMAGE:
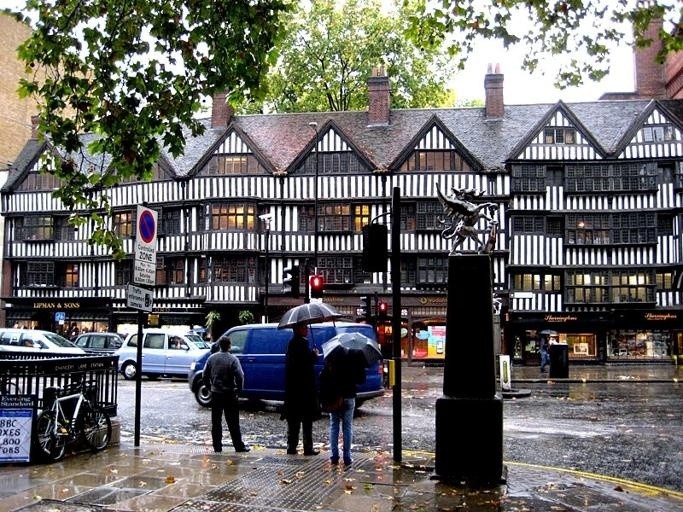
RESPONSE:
[215,448,353,465]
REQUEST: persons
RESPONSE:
[171,336,186,350]
[538,337,550,373]
[317,348,357,467]
[319,331,383,370]
[279,322,324,456]
[200,335,251,454]
[5,319,111,351]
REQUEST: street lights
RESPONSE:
[256,212,273,324]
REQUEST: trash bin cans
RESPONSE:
[549,344,570,379]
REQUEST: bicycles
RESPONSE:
[33,370,112,462]
[0,372,22,396]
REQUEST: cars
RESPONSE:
[109,330,211,379]
[71,333,136,371]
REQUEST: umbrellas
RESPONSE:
[276,302,343,329]
[539,329,559,335]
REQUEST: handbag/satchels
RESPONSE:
[322,396,343,413]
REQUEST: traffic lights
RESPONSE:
[377,302,387,320]
[359,295,371,320]
[307,274,324,299]
[282,264,299,301]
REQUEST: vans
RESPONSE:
[186,322,386,409]
[0,327,85,356]
